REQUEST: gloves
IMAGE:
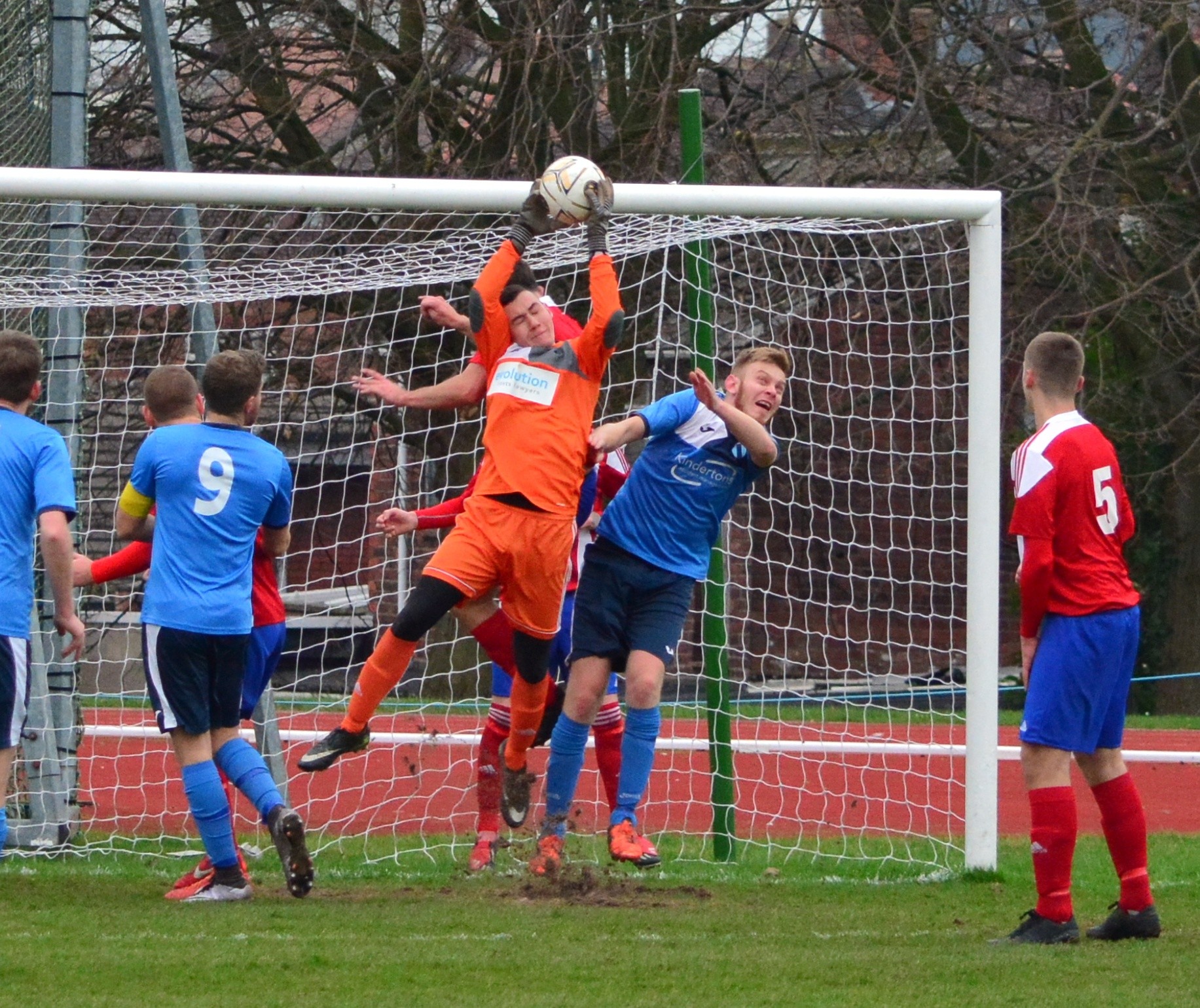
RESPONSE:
[504,178,572,245]
[585,174,613,251]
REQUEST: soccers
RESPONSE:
[539,155,607,225]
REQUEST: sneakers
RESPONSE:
[498,738,536,827]
[182,881,250,903]
[529,835,563,875]
[469,829,500,870]
[631,834,663,869]
[164,868,252,901]
[1085,900,1162,942]
[272,808,315,897]
[298,720,370,770]
[174,849,248,889]
[607,819,642,862]
[987,909,1079,946]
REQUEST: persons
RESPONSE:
[527,347,793,877]
[375,448,661,877]
[73,365,285,899]
[298,176,624,829]
[1,329,85,860]
[1010,332,1162,943]
[115,349,315,903]
[353,260,584,744]
